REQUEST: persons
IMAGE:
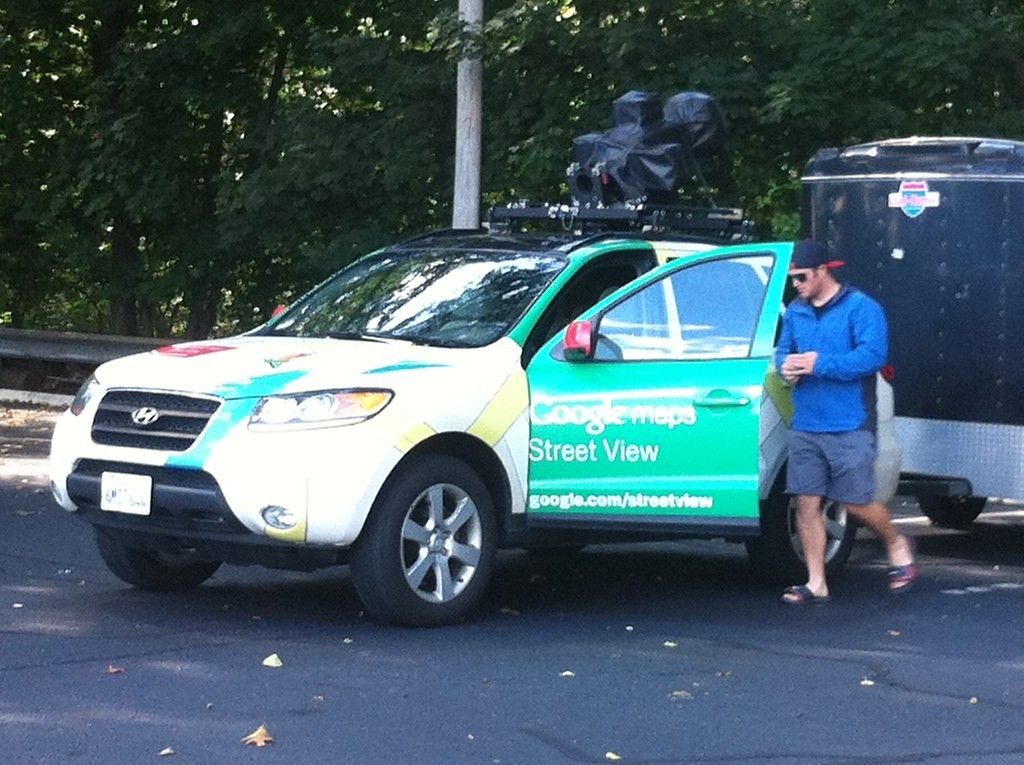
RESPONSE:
[774,240,920,605]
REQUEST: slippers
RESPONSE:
[780,583,831,605]
[887,531,919,594]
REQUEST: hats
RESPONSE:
[788,235,846,268]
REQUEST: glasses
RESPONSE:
[791,270,815,283]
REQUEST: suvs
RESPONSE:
[46,208,904,632]
